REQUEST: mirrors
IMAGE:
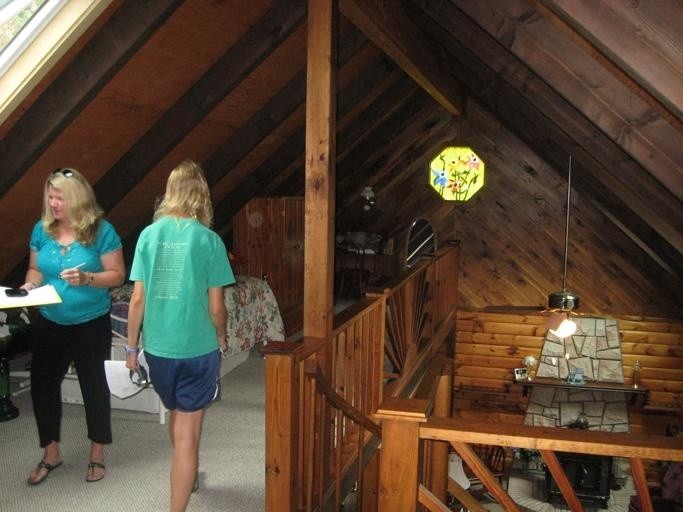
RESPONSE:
[403,215,438,276]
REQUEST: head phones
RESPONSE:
[129,362,148,387]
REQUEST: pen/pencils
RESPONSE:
[58,261,87,276]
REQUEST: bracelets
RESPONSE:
[123,344,138,354]
[25,281,39,289]
[86,269,94,286]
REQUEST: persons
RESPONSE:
[25,168,126,484]
[126,160,236,508]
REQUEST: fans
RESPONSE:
[483,155,622,333]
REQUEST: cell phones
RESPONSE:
[6,288,28,296]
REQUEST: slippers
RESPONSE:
[86,462,106,482]
[29,460,62,484]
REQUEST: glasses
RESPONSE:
[130,366,148,387]
[53,168,72,178]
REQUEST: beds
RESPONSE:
[60,275,266,425]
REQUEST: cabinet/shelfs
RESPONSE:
[233,197,303,338]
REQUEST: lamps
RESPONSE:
[359,185,376,213]
[548,319,575,341]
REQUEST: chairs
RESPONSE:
[470,444,509,493]
[335,239,369,302]
